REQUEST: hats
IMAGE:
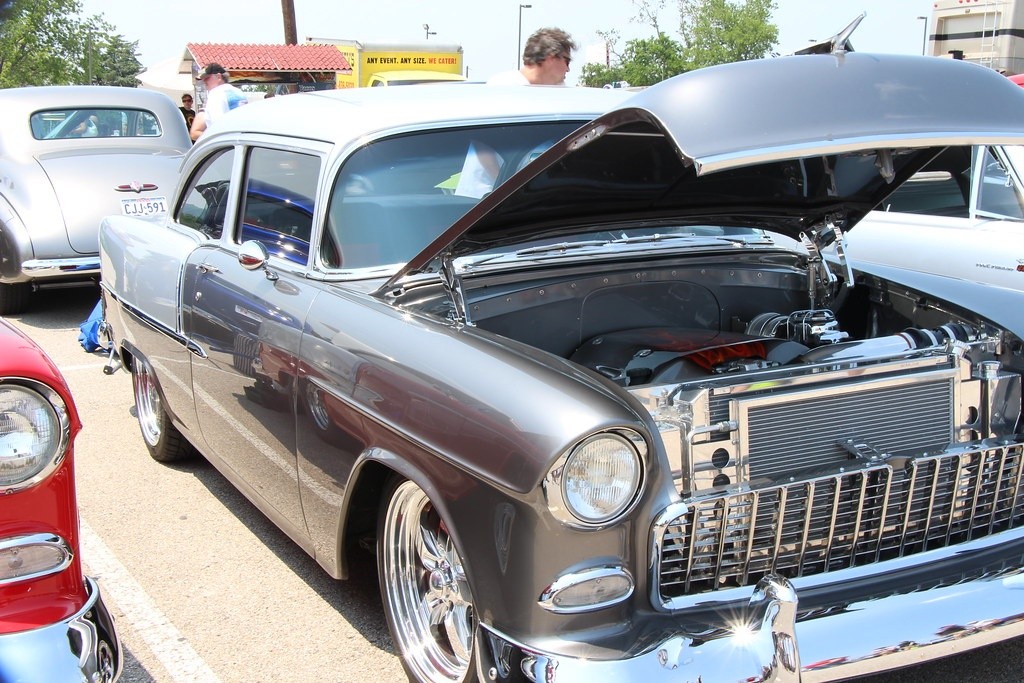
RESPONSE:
[195,61,225,80]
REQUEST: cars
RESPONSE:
[762,146,1024,343]
[92,11,1024,683]
[134,174,345,348]
[0,314,129,683]
[0,85,207,317]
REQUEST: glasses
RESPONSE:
[183,98,193,103]
[554,53,571,67]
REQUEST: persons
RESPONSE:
[455,27,577,199]
[195,62,248,127]
[178,94,197,145]
[65,108,99,138]
[189,99,207,141]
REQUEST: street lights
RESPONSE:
[518,3,532,70]
[916,15,928,57]
[422,24,437,40]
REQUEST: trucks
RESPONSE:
[306,33,470,90]
